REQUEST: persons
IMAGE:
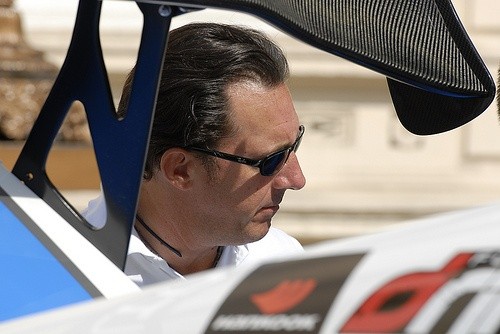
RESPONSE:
[80,21,311,293]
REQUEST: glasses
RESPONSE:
[170,126,305,178]
